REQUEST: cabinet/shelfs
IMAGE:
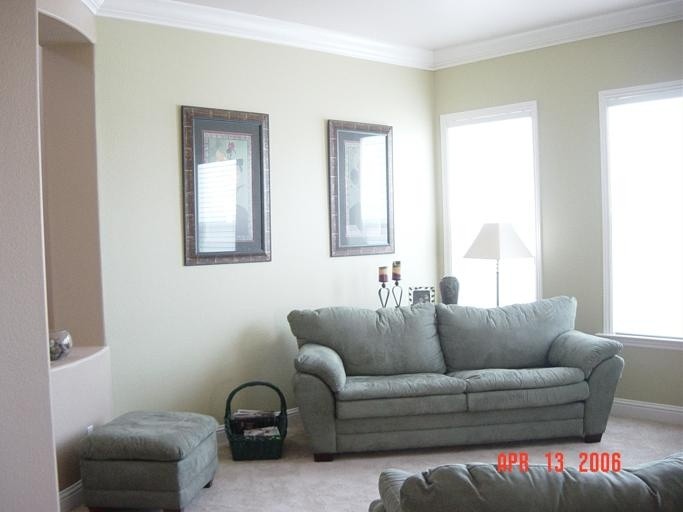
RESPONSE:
[0,0,115,512]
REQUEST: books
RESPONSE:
[228,408,282,439]
[56,476,83,511]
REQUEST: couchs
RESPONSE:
[292,331,624,462]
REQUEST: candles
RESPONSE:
[379,267,388,284]
[392,261,401,279]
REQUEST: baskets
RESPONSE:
[225,382,287,461]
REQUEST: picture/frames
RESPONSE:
[181,105,274,266]
[407,286,435,304]
[328,119,396,257]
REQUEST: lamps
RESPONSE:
[464,223,535,307]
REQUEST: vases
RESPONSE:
[439,276,459,304]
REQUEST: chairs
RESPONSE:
[369,469,412,512]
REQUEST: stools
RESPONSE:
[81,411,218,512]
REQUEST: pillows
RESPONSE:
[287,303,446,375]
[437,296,578,370]
[401,452,683,512]
